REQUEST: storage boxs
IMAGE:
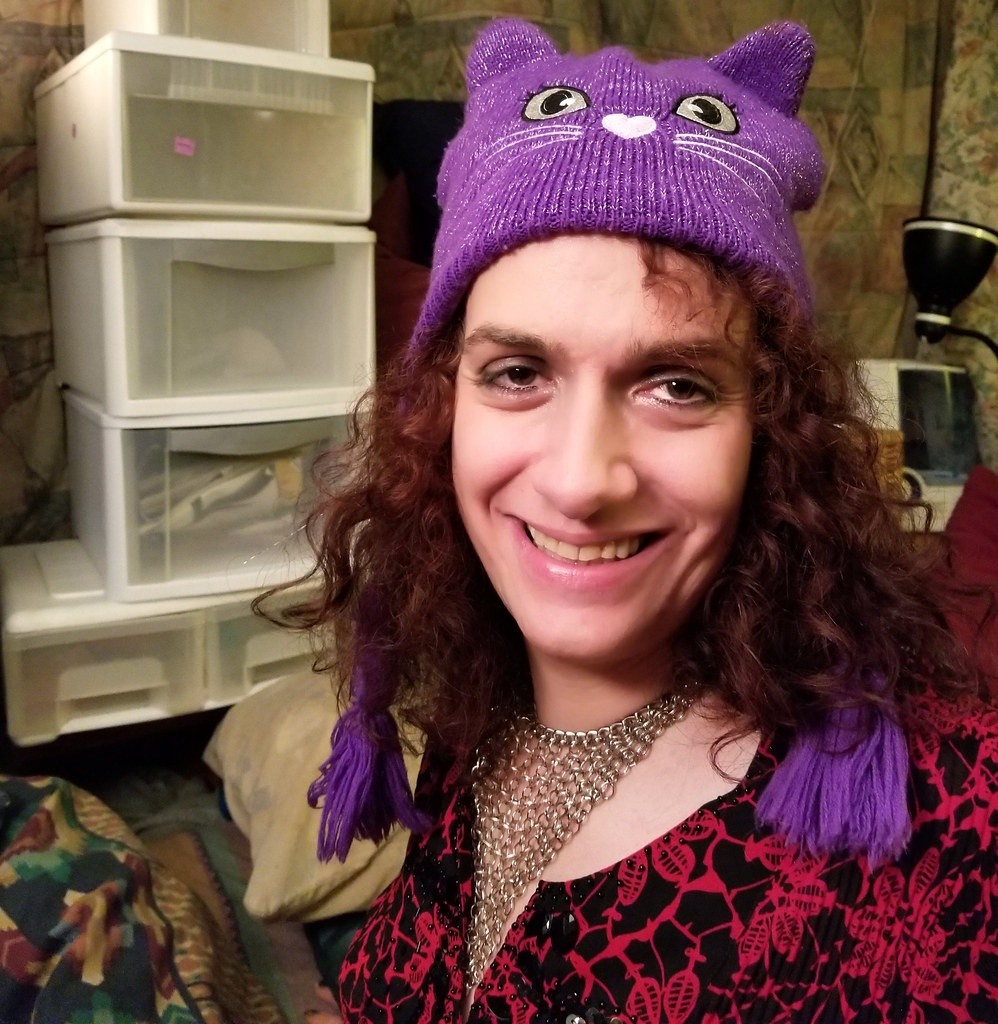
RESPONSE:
[0,0,378,748]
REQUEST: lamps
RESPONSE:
[900,216,997,363]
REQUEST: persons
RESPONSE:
[305,19,998,1024]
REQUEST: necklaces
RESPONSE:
[466,676,701,991]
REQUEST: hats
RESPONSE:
[309,21,912,872]
